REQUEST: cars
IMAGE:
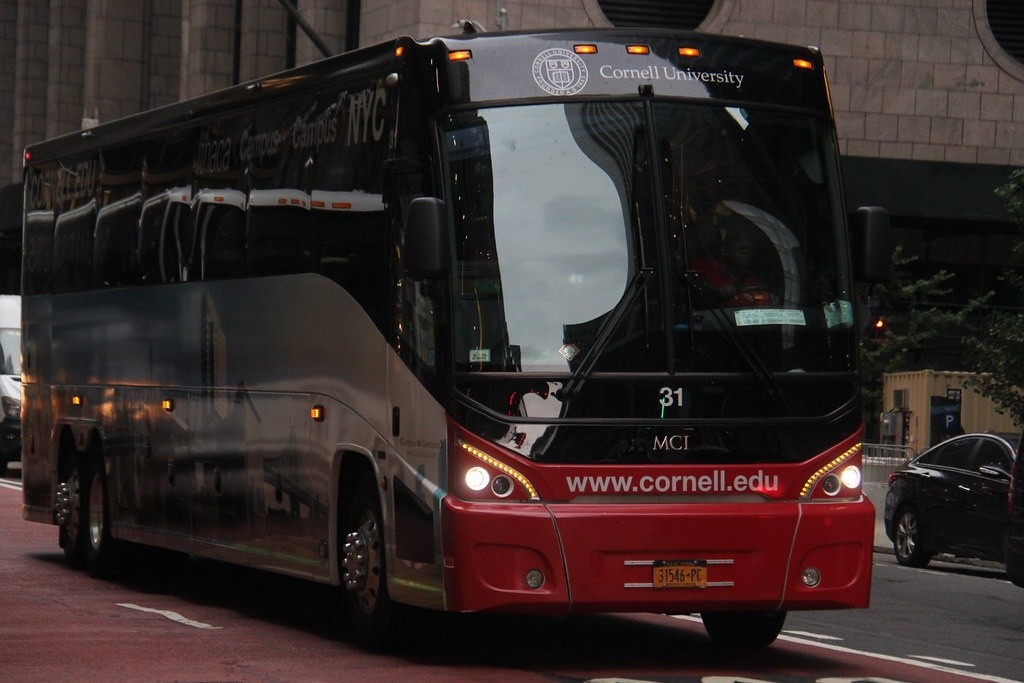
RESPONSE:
[883,432,1022,567]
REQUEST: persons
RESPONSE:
[692,227,770,307]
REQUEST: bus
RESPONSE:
[21,21,891,651]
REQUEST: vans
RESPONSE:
[0,295,22,472]
